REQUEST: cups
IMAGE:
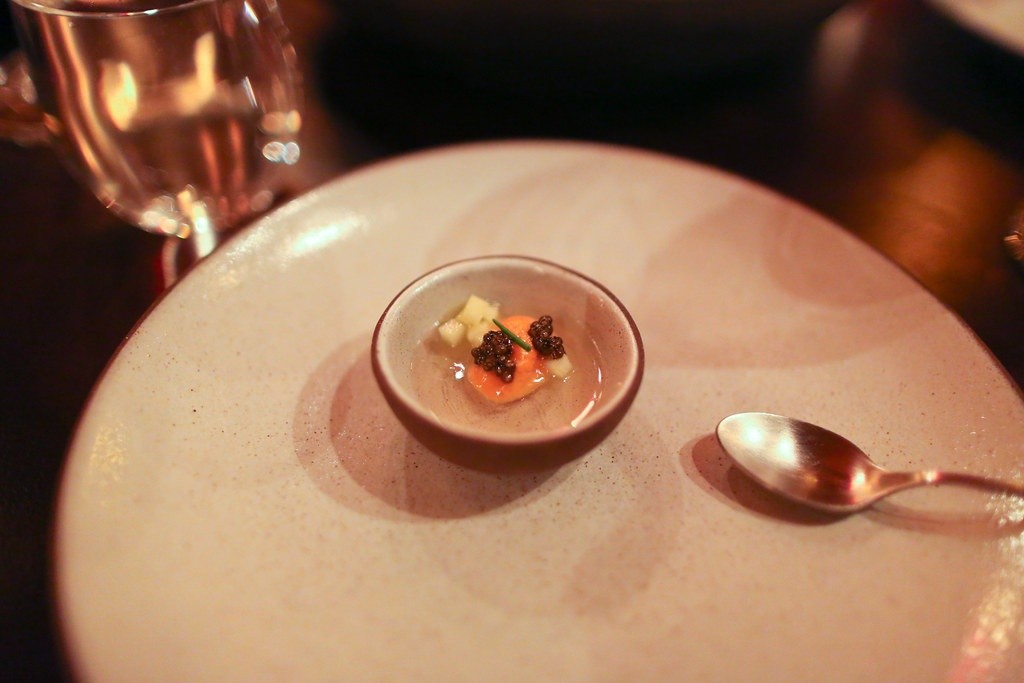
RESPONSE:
[0,0,308,238]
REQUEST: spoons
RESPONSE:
[715,413,1024,560]
[57,140,1024,683]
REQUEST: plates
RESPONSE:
[370,255,645,474]
